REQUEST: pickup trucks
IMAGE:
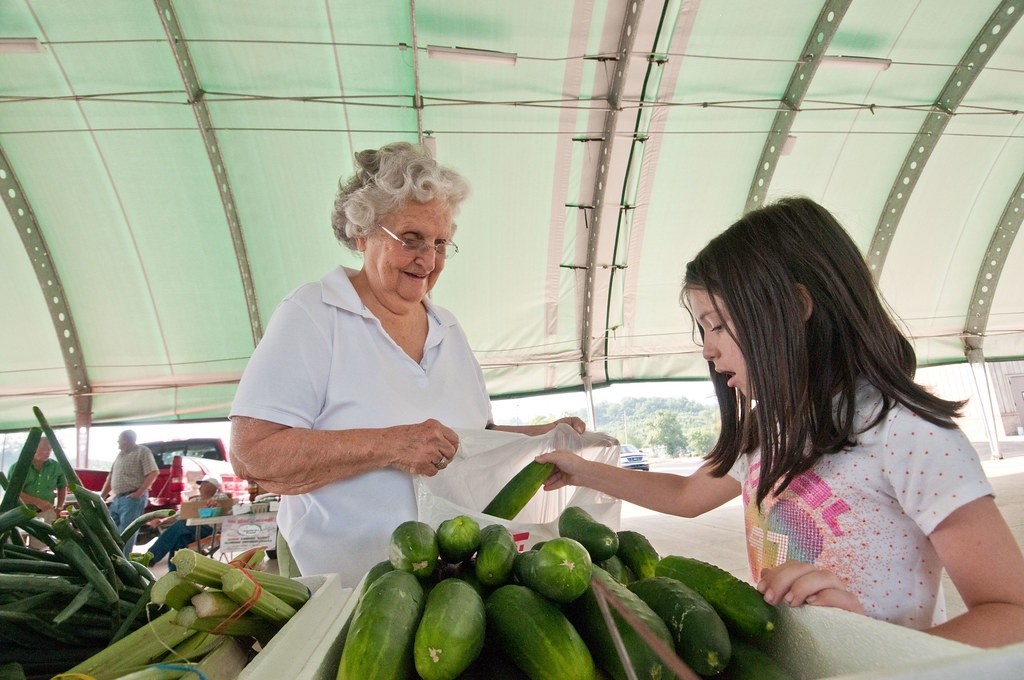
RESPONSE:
[64,438,249,515]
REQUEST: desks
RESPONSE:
[186,511,279,560]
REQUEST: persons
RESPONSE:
[145,475,228,571]
[536,198,1023,649]
[101,430,160,561]
[228,141,619,585]
[7,437,68,550]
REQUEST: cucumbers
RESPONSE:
[482,459,555,520]
[332,506,799,680]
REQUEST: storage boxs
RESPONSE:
[230,570,352,680]
[295,571,1024,680]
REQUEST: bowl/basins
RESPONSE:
[212,507,221,516]
[197,508,215,516]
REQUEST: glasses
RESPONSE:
[377,223,458,259]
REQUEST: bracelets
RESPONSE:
[57,508,63,510]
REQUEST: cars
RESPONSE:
[247,479,282,560]
[620,444,650,472]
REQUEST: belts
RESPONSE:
[117,490,135,497]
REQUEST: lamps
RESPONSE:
[820,55,891,70]
[0,39,46,55]
[422,135,437,161]
[425,45,517,66]
[782,135,798,158]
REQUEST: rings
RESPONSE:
[435,457,446,468]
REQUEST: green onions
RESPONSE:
[0,406,176,680]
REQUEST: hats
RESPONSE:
[195,478,220,489]
